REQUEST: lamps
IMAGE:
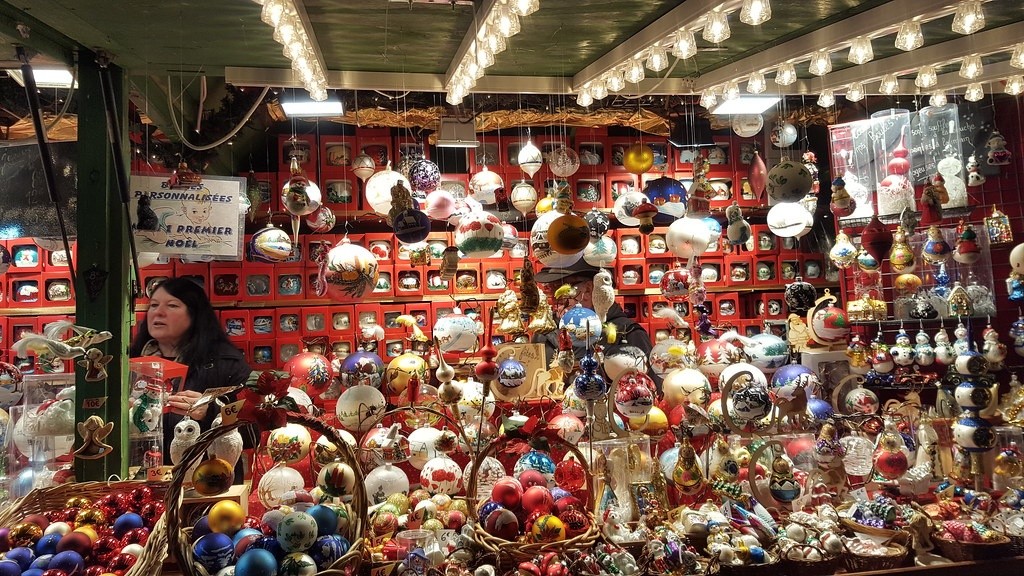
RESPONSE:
[251,0,1024,148]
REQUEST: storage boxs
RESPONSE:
[0,235,79,380]
[135,134,845,391]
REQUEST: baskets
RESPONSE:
[0,406,1024,576]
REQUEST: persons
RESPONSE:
[529,254,665,401]
[129,277,256,484]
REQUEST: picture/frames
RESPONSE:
[128,171,246,261]
